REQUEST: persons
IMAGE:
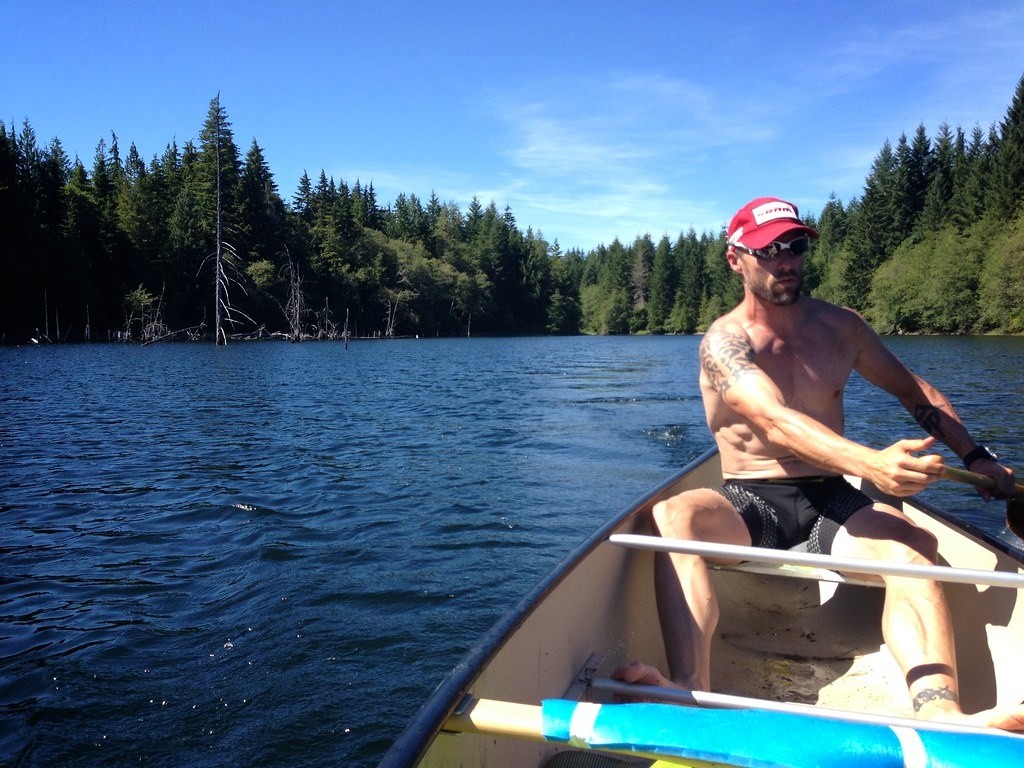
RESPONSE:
[609,197,1024,733]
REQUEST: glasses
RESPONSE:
[735,235,814,257]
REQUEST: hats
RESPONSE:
[725,196,819,249]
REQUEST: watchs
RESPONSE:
[963,445,997,471]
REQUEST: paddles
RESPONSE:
[919,458,1024,545]
